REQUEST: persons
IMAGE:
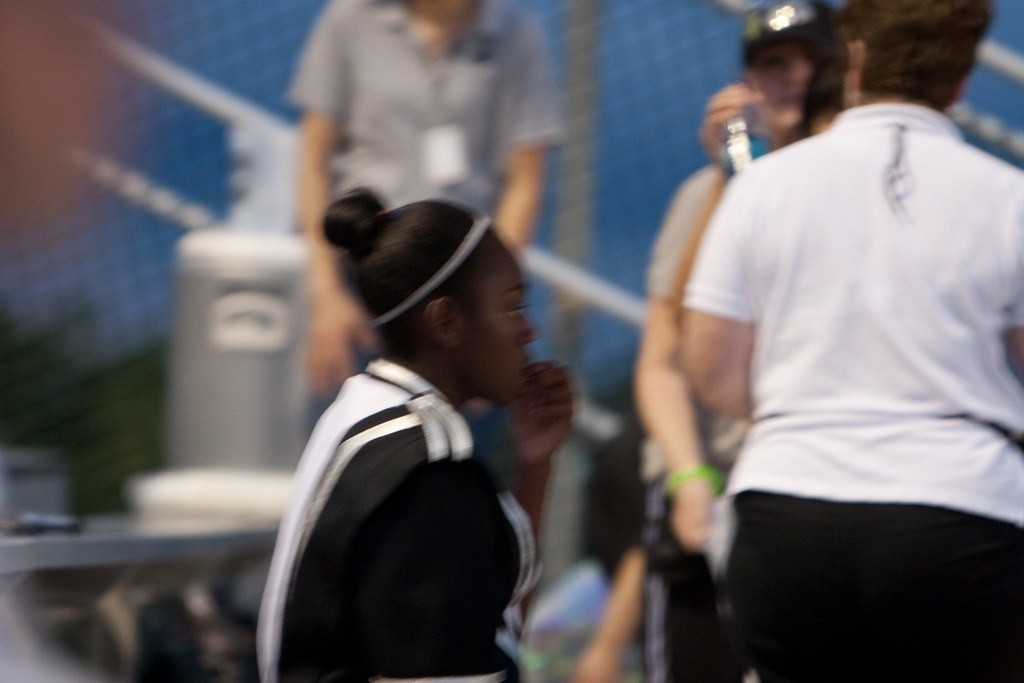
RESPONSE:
[291,0,568,433]
[254,186,571,683]
[577,0,1023,683]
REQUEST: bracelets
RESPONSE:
[666,465,724,495]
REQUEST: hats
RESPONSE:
[741,0,837,69]
